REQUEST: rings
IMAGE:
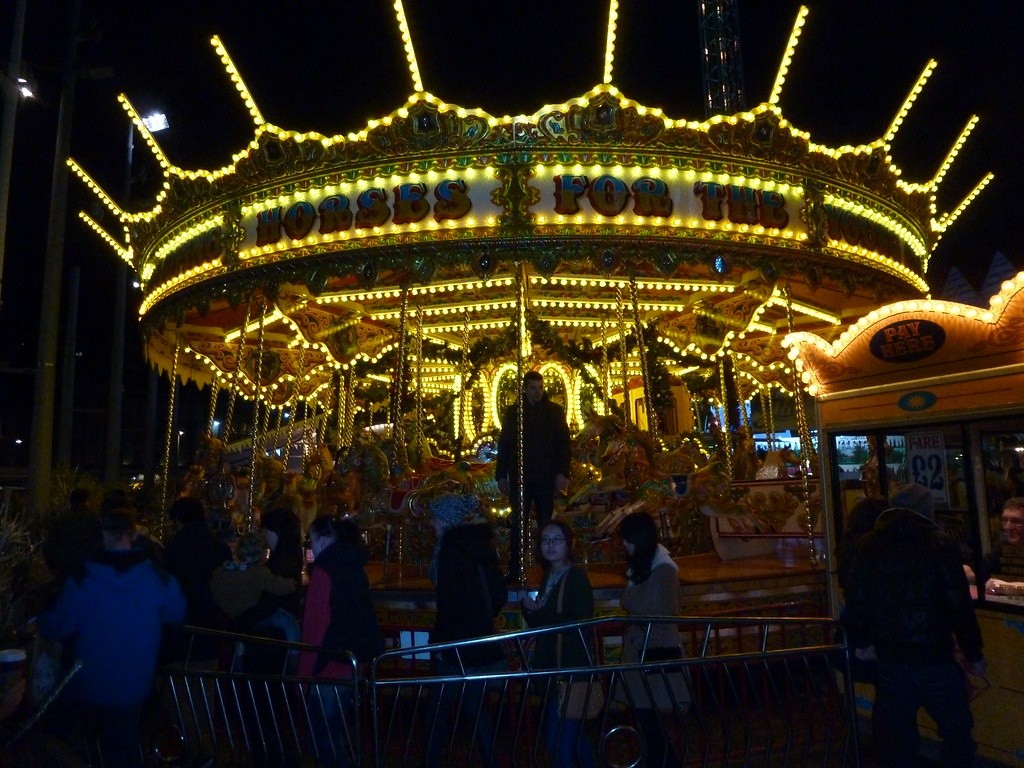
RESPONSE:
[992,589,994,592]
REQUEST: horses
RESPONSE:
[183,407,830,583]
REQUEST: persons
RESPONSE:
[613,511,693,768]
[298,514,370,768]
[427,496,509,768]
[519,519,594,768]
[210,532,301,768]
[158,496,233,768]
[256,492,304,579]
[963,498,1024,595]
[834,482,988,768]
[38,508,187,768]
[43,489,165,576]
[495,371,571,584]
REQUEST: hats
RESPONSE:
[891,483,933,512]
[431,494,480,525]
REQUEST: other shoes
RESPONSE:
[504,569,518,584]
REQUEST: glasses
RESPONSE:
[541,537,569,546]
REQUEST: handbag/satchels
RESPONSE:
[558,681,603,720]
[637,646,681,674]
